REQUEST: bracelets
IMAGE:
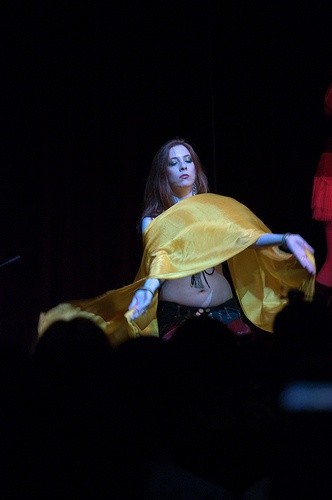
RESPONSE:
[138,287,153,297]
[282,232,289,245]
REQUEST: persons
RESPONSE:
[126,141,317,341]
[310,82,332,307]
[0,302,332,500]
